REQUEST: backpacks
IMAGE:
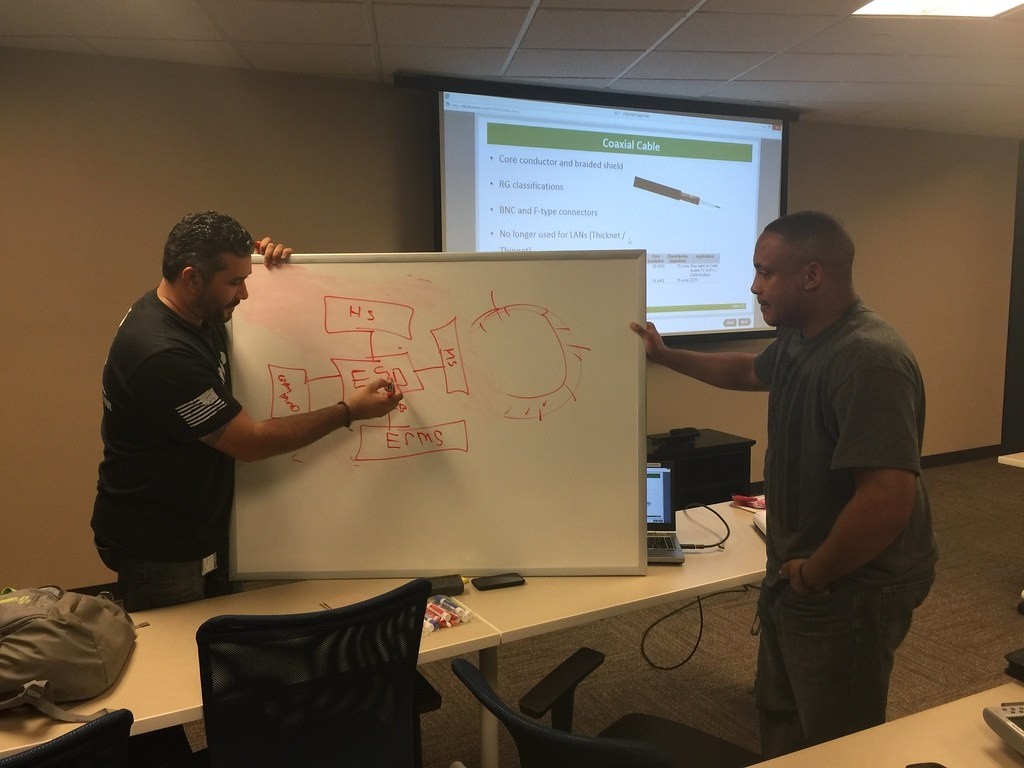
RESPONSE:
[0,585,149,723]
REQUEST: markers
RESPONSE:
[421,595,473,633]
[387,377,395,400]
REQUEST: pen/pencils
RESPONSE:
[632,175,723,211]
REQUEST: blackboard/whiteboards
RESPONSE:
[225,244,650,581]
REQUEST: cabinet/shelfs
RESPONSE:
[647,428,757,510]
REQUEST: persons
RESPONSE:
[90,210,403,768]
[631,211,936,760]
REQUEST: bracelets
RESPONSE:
[337,401,351,427]
[800,562,827,593]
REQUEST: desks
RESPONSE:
[0,577,501,768]
[450,493,767,644]
[747,680,1024,767]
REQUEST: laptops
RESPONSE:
[646,460,684,564]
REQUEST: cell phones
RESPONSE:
[472,573,525,591]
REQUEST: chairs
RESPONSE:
[160,578,443,767]
[0,706,159,767]
[450,646,766,768]
